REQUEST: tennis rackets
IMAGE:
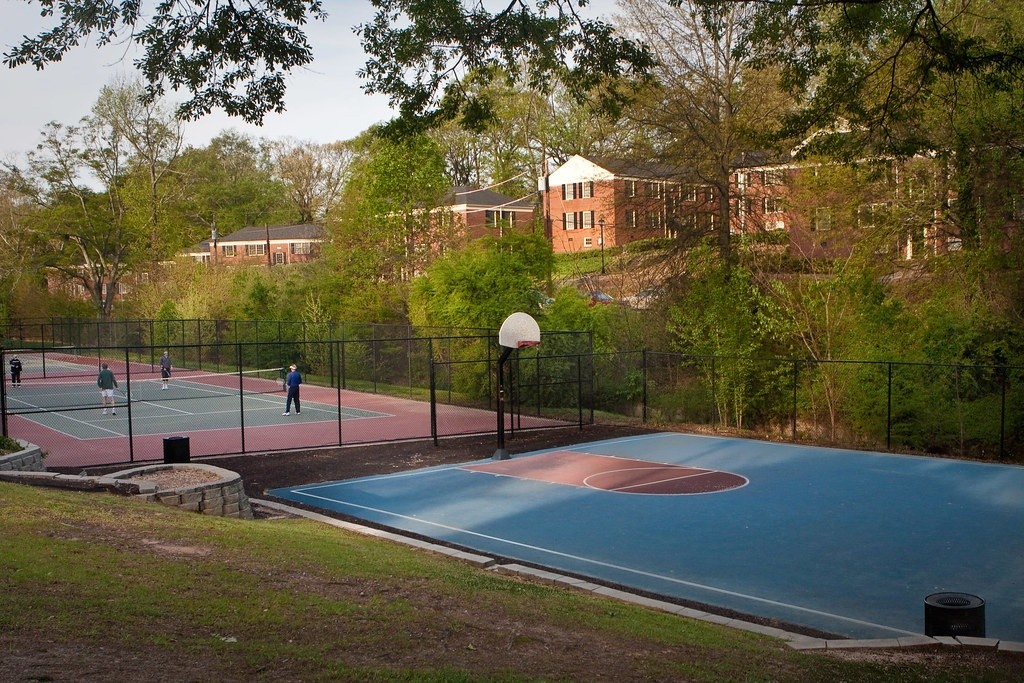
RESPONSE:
[119,390,134,398]
[276,377,291,388]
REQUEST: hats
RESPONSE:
[290,365,297,369]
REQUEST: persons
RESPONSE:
[97,363,119,415]
[281,365,302,415]
[160,351,173,390]
[9,355,23,387]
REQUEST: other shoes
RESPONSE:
[166,388,168,389]
[282,413,289,415]
[102,411,107,414]
[163,388,165,389]
[18,386,21,389]
[295,412,300,414]
[113,410,116,415]
[12,386,16,388]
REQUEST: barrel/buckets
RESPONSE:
[925,593,986,637]
[163,435,191,464]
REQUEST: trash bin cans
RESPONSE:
[163,436,191,465]
[923,591,986,638]
